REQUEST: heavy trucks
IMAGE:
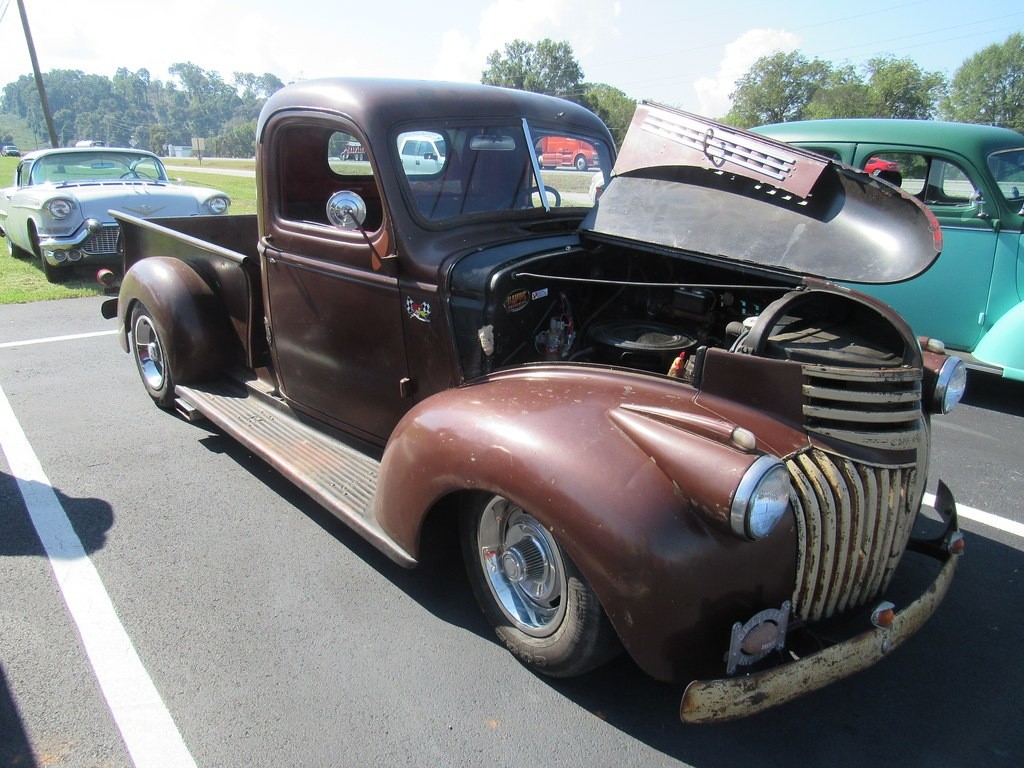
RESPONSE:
[339,140,369,163]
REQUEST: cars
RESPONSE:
[0,146,232,285]
[1,145,21,157]
[99,71,976,728]
[739,119,1024,397]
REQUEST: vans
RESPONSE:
[397,132,446,176]
[536,134,600,172]
